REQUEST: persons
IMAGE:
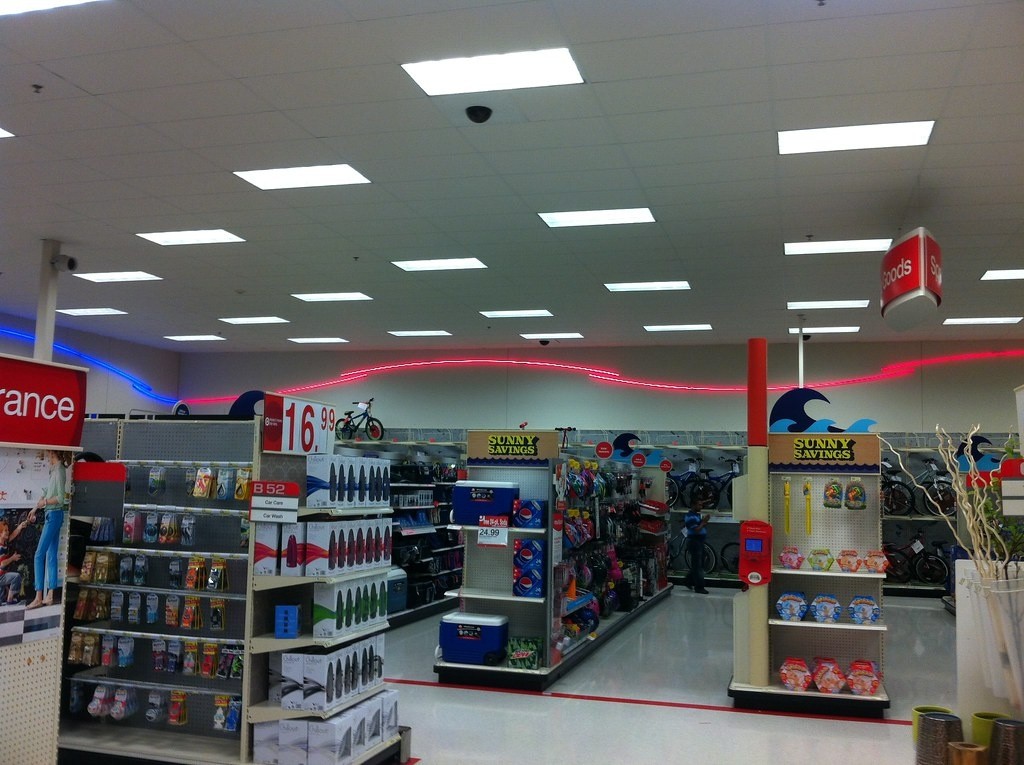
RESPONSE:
[0,518,28,605]
[24,450,67,610]
[680,497,710,595]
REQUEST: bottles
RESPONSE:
[447,550,461,569]
[458,530,463,544]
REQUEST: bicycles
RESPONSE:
[721,542,740,573]
[665,456,743,508]
[883,532,952,584]
[667,525,717,574]
[336,398,384,440]
[881,457,956,516]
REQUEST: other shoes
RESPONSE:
[42,599,53,605]
[684,583,693,589]
[6,600,19,606]
[26,600,42,609]
[695,587,709,594]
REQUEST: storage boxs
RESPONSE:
[254,522,282,576]
[281,646,323,710]
[355,696,382,750]
[307,452,391,509]
[281,522,307,576]
[304,518,392,712]
[508,635,547,670]
[439,612,508,665]
[513,571,548,598]
[513,499,548,528]
[513,539,548,570]
[269,648,296,701]
[336,706,366,759]
[374,688,399,742]
[253,713,353,765]
[387,565,407,615]
[452,480,520,528]
[275,602,304,638]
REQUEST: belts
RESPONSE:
[47,507,62,510]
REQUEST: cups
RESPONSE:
[970,711,1013,745]
[914,713,963,765]
[989,718,1024,765]
[912,705,953,752]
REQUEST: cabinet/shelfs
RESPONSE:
[668,507,956,598]
[434,462,552,692]
[387,483,465,633]
[728,471,891,719]
[57,419,401,765]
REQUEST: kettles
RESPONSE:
[440,508,454,524]
[448,573,458,588]
